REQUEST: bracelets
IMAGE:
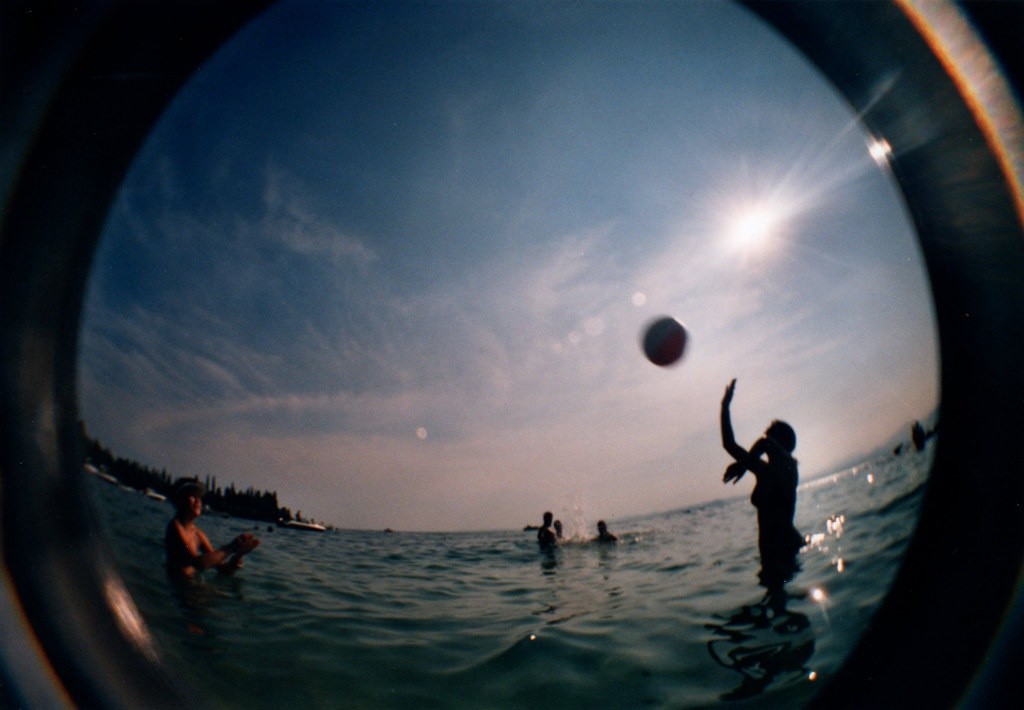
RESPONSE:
[231,557,244,570]
[221,544,232,555]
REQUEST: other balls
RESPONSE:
[636,313,688,368]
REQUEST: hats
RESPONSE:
[172,478,205,497]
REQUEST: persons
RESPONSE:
[721,379,805,618]
[553,519,565,543]
[591,520,618,542]
[165,477,259,593]
[538,512,556,544]
[912,420,926,453]
